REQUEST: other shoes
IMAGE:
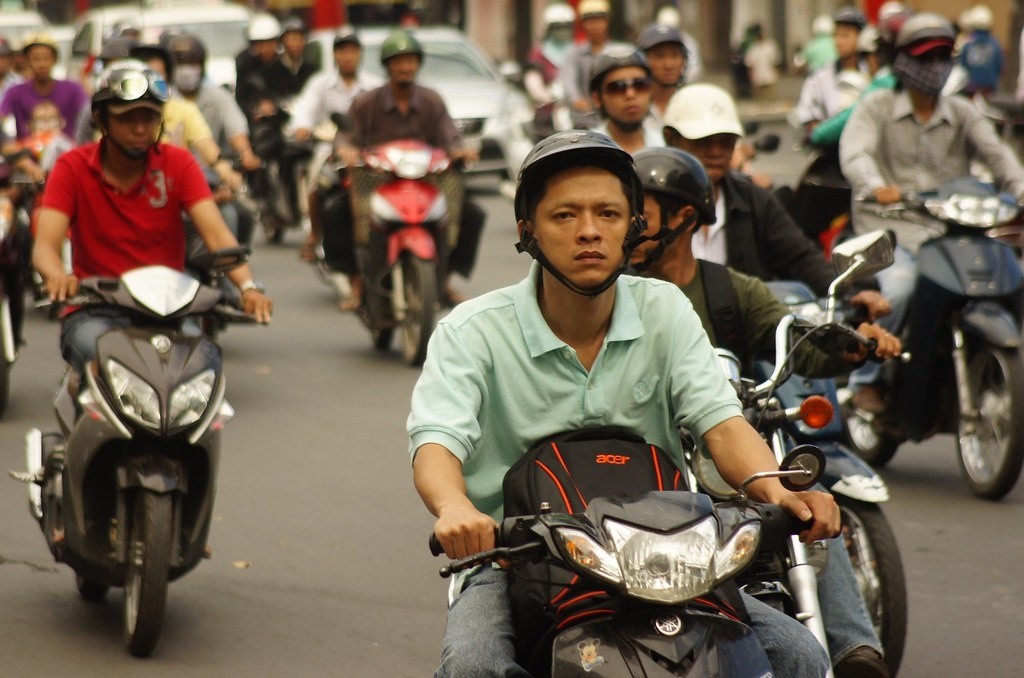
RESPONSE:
[441,286,468,308]
[852,384,887,413]
[298,241,362,312]
[836,646,890,678]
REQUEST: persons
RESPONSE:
[29,60,277,562]
[0,14,488,351]
[630,147,902,678]
[523,0,1024,415]
[403,129,842,678]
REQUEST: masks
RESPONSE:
[892,52,952,95]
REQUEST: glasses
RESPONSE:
[111,71,169,102]
[602,76,648,93]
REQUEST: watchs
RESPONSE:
[239,282,265,293]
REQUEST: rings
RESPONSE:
[878,301,884,306]
[885,334,890,338]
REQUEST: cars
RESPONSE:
[311,28,526,185]
[0,0,256,106]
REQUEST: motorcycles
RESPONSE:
[707,230,908,678]
[22,265,261,661]
[310,110,487,367]
[429,443,841,678]
[827,180,1024,501]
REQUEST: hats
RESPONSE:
[663,83,744,140]
[107,97,164,117]
[637,25,684,50]
[910,38,957,55]
[334,32,363,49]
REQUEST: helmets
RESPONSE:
[91,57,167,103]
[959,5,993,31]
[812,1,907,57]
[0,28,59,59]
[589,46,651,88]
[243,13,308,44]
[514,129,645,217]
[543,0,611,34]
[895,13,955,53]
[381,33,423,64]
[101,28,205,81]
[631,147,717,225]
